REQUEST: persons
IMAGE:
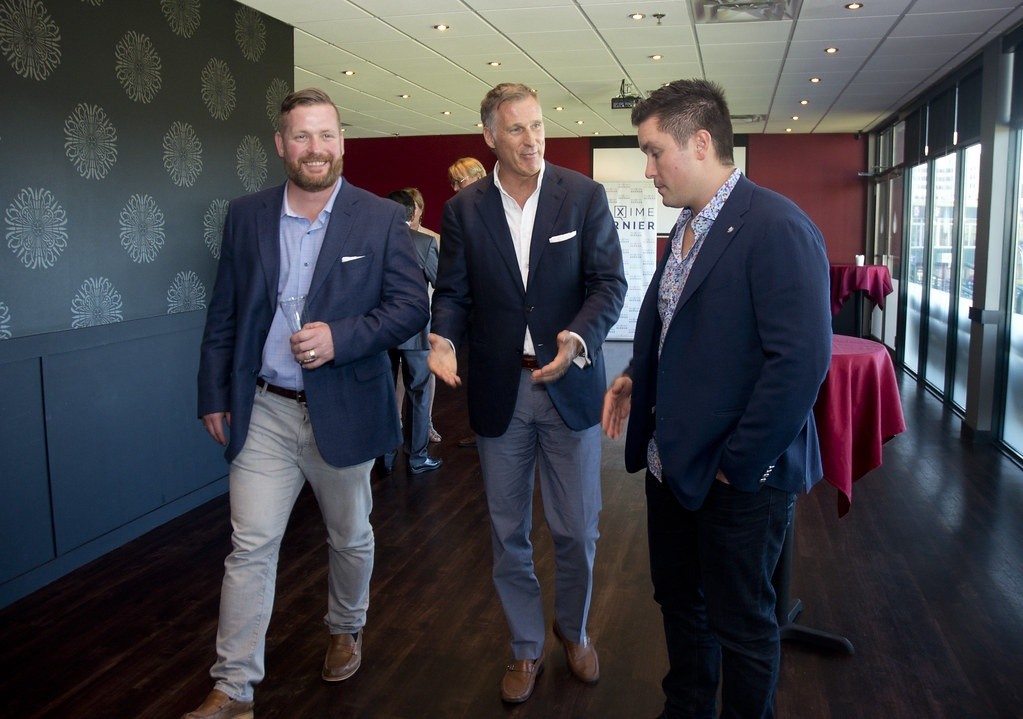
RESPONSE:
[182,88,430,719]
[599,79,833,719]
[425,82,629,703]
[449,158,487,192]
[378,188,441,475]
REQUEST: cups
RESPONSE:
[855,255,864,266]
[280,296,317,363]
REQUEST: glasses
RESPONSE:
[451,176,469,188]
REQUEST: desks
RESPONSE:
[828,263,894,338]
[813,333,907,520]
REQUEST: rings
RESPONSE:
[309,350,315,358]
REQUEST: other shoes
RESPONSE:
[458,436,479,446]
[428,418,443,442]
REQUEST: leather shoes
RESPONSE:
[501,651,546,701]
[381,465,392,475]
[178,690,254,719]
[410,456,442,474]
[320,628,363,681]
[552,626,600,683]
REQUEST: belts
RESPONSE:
[521,357,539,371]
[255,377,308,403]
